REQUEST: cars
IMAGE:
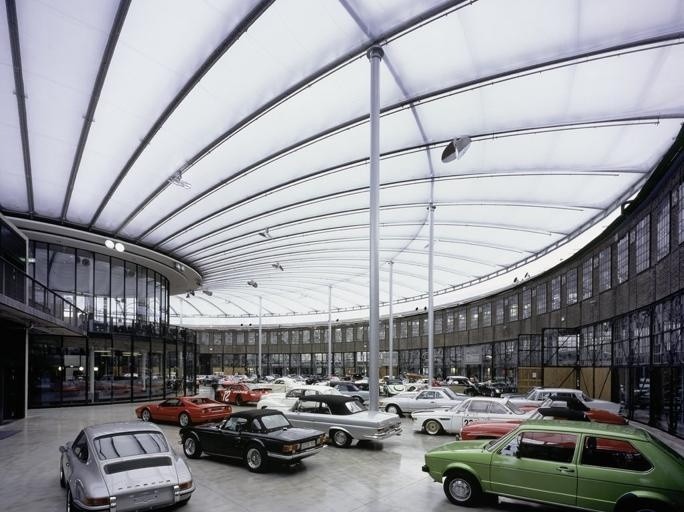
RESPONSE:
[37,369,191,397]
[420,418,684,512]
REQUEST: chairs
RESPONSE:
[585,437,599,465]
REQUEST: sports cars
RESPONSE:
[52,417,197,512]
[134,394,232,427]
[179,405,329,475]
